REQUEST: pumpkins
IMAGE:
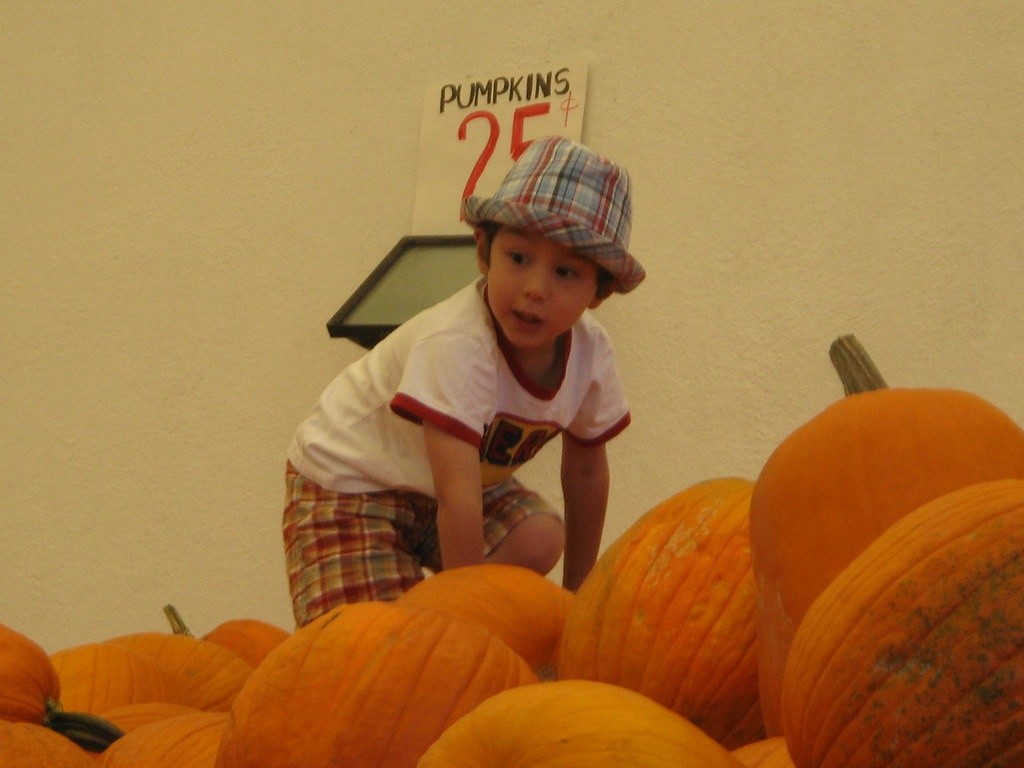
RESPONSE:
[0,331,1024,768]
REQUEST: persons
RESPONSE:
[282,136,645,631]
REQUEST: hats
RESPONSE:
[461,136,647,295]
